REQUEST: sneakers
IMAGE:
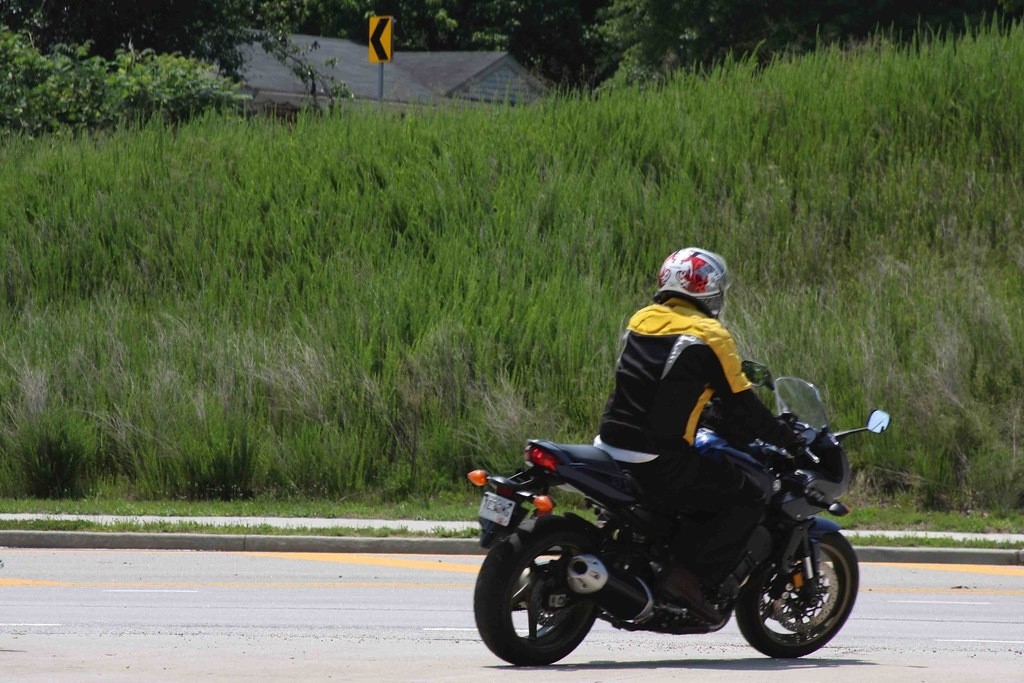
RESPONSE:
[662,569,721,627]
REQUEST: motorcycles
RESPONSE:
[468,360,893,668]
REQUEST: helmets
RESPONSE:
[651,247,727,317]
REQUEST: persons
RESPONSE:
[593,246,809,622]
